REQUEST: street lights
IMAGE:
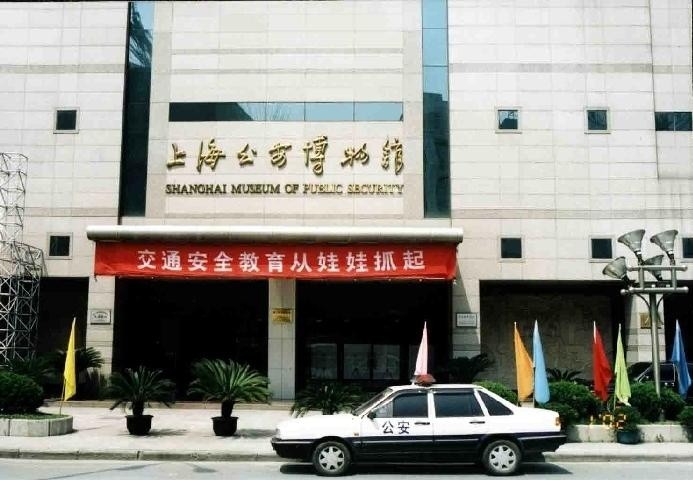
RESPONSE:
[601,229,688,422]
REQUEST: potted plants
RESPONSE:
[100,358,273,436]
[614,406,642,444]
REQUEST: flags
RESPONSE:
[514,325,535,403]
[415,324,428,375]
[592,324,613,402]
[533,322,550,403]
[614,327,631,406]
[670,321,692,394]
[62,320,76,402]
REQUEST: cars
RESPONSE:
[578,362,693,414]
[271,374,569,476]
